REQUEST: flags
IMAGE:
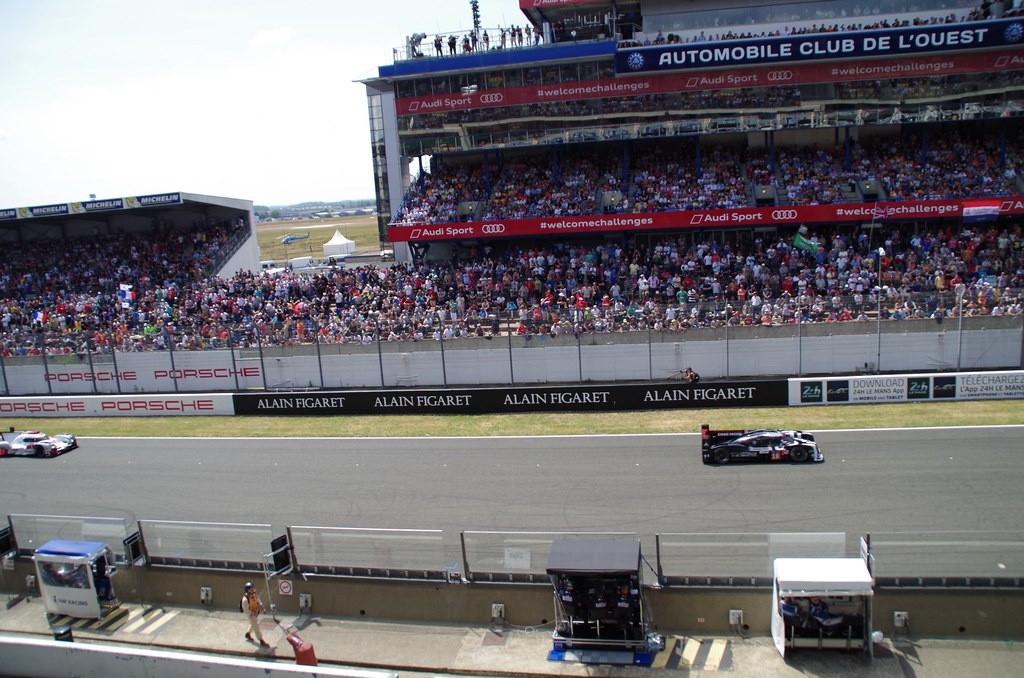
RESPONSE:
[793,232,819,250]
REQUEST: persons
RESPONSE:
[558,574,642,633]
[242,582,269,647]
[809,596,831,635]
[73,557,117,601]
[779,596,803,635]
[42,562,67,587]
[680,366,696,383]
[0,0,1024,354]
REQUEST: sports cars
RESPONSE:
[701,424,823,464]
[0,427,78,458]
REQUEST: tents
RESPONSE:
[323,229,355,255]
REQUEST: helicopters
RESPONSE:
[276,232,310,245]
[331,215,340,218]
[311,215,320,219]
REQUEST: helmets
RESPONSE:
[245,582,253,593]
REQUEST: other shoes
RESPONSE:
[245,632,254,642]
[260,640,270,649]
